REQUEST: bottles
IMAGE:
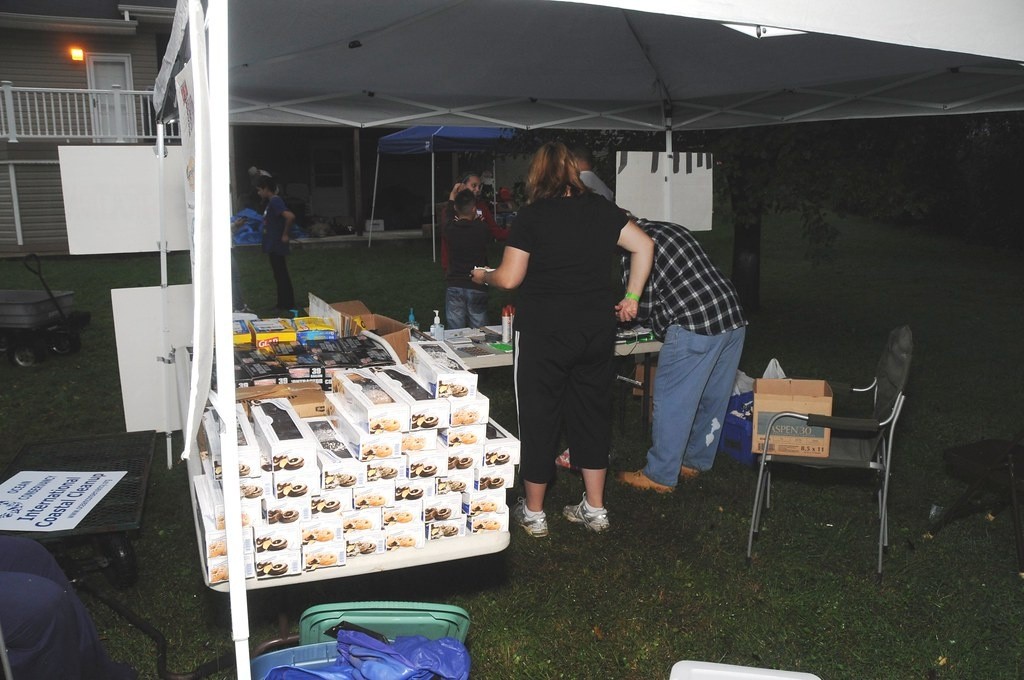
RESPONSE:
[502,305,515,343]
[408,308,415,323]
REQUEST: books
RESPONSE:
[443,326,514,357]
[307,292,357,337]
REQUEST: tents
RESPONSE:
[156,0,1024,680]
[368,126,517,261]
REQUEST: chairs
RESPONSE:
[745,323,916,575]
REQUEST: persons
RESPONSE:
[0,534,137,680]
[469,140,653,537]
[253,177,295,312]
[231,187,249,311]
[442,188,494,329]
[616,207,749,494]
[439,172,510,271]
[569,141,613,201]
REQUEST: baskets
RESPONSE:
[718,391,760,465]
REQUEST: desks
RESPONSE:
[189,476,517,609]
[0,429,187,680]
[434,315,663,445]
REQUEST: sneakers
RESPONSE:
[514,496,549,537]
[680,465,699,479]
[563,491,609,532]
[614,469,672,493]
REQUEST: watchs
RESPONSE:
[625,292,640,302]
[482,270,490,287]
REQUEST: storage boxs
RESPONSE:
[752,378,834,456]
[720,390,755,464]
[250,638,463,680]
[195,300,520,587]
